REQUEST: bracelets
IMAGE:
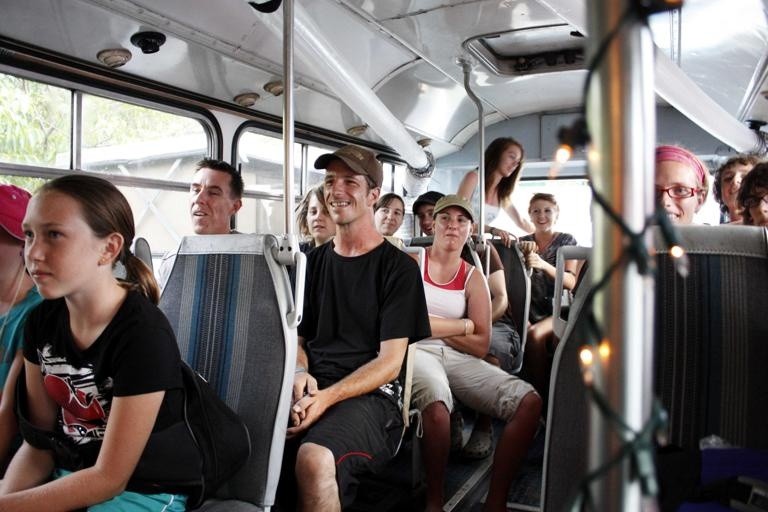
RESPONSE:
[462,318,467,337]
[293,368,307,373]
[462,317,469,337]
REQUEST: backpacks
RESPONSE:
[15,364,251,504]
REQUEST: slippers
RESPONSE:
[464,424,494,460]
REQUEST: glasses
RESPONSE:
[653,185,704,199]
[739,191,768,209]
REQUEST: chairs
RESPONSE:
[488,231,534,374]
[409,234,493,360]
[155,235,297,512]
[539,223,767,511]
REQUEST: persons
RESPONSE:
[656,145,708,224]
[712,157,756,224]
[411,189,521,458]
[286,144,412,512]
[3,174,205,511]
[737,161,768,227]
[375,194,404,236]
[457,137,533,248]
[0,186,46,398]
[156,159,244,294]
[407,195,542,510]
[298,186,336,255]
[515,193,577,379]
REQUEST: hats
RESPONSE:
[413,191,443,214]
[314,147,384,189]
[1,184,32,241]
[433,195,475,223]
